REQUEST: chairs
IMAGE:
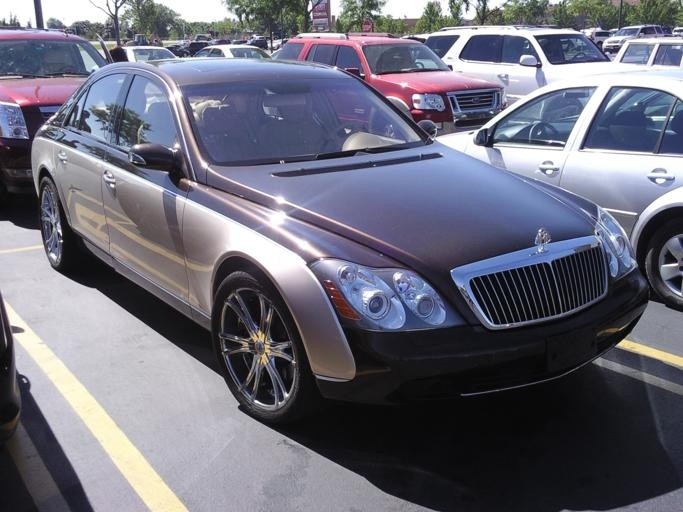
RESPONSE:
[148,102,336,163]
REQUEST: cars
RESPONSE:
[1,26,109,201]
[432,72,683,310]
[31,55,649,428]
[104,33,288,65]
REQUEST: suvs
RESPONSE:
[424,24,610,105]
[579,24,683,69]
[270,35,508,136]
